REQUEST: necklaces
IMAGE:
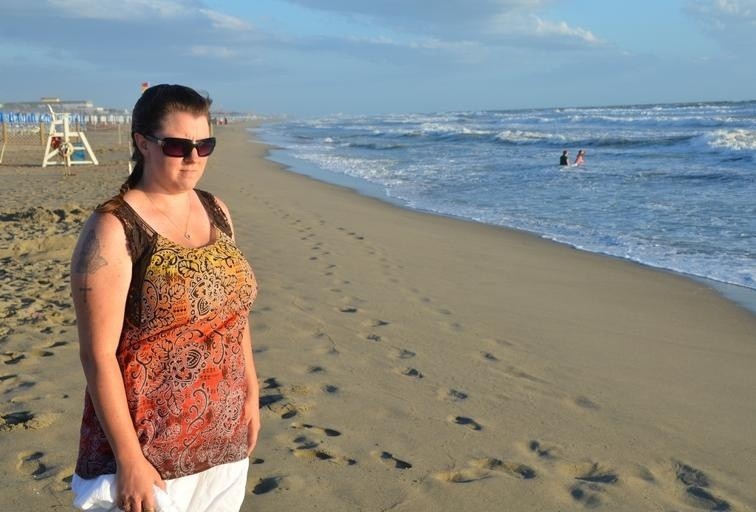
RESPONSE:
[141,187,194,241]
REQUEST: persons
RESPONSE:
[574,151,585,164]
[65,85,261,512]
[560,151,570,166]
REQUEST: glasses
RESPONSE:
[143,134,216,157]
